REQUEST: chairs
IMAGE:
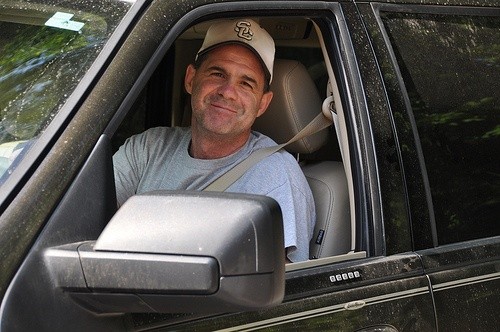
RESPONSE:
[250,51,352,278]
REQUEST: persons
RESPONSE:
[112,17,316,265]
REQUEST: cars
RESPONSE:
[1,0,500,331]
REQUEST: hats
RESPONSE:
[194,20,277,92]
